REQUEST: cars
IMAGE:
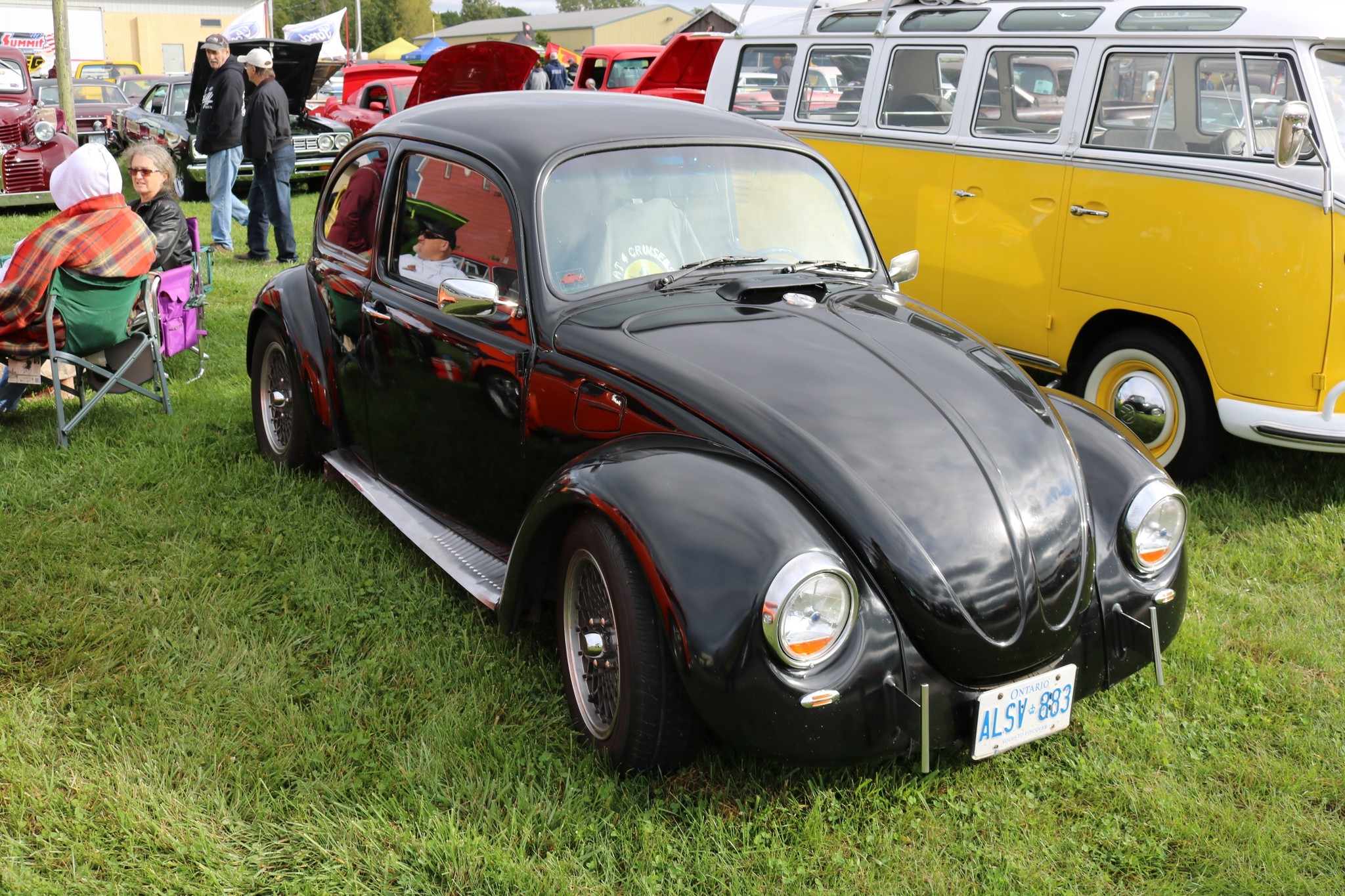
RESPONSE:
[109,75,190,108]
[245,89,1191,789]
[122,38,359,202]
[564,44,1291,138]
[71,60,168,103]
[28,78,133,152]
[0,45,80,208]
[301,40,541,135]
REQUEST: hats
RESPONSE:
[200,33,228,51]
[423,219,457,248]
[237,48,273,68]
[550,52,558,60]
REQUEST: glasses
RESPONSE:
[244,65,251,69]
[127,168,161,177]
[418,229,447,243]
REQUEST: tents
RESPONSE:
[509,31,547,57]
[400,36,450,62]
[366,36,418,61]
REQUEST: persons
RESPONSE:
[769,51,809,111]
[22,140,195,401]
[235,47,300,265]
[1145,71,1159,92]
[326,149,469,289]
[191,33,271,254]
[521,52,580,91]
[0,143,157,416]
[1199,71,1216,91]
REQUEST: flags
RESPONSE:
[217,0,268,41]
[281,7,348,60]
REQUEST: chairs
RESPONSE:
[896,93,952,126]
[131,216,215,389]
[1102,128,1188,152]
[1208,127,1278,156]
[0,263,173,451]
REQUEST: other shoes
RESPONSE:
[245,222,271,245]
[209,242,233,255]
[265,259,299,266]
[233,253,270,263]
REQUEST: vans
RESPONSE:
[698,1,1344,490]
